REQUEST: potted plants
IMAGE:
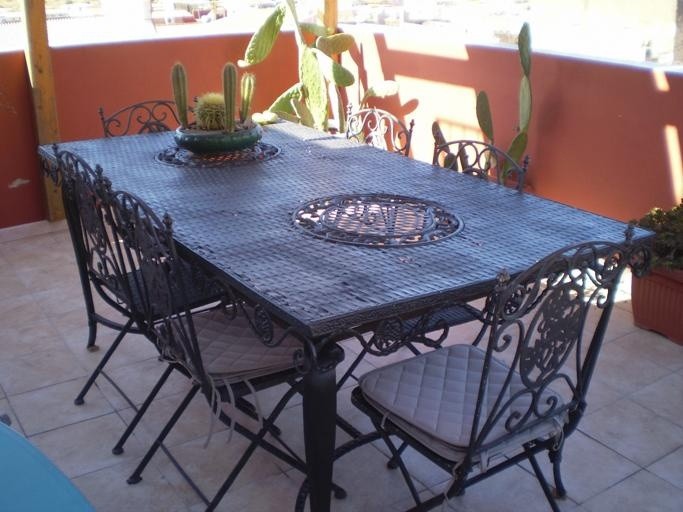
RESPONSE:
[170,62,263,155]
[630,198,683,345]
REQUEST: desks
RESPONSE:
[37,118,635,511]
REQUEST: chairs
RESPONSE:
[93,100,207,138]
[329,134,534,393]
[89,178,353,502]
[50,139,235,411]
[340,101,416,158]
[360,233,657,512]
